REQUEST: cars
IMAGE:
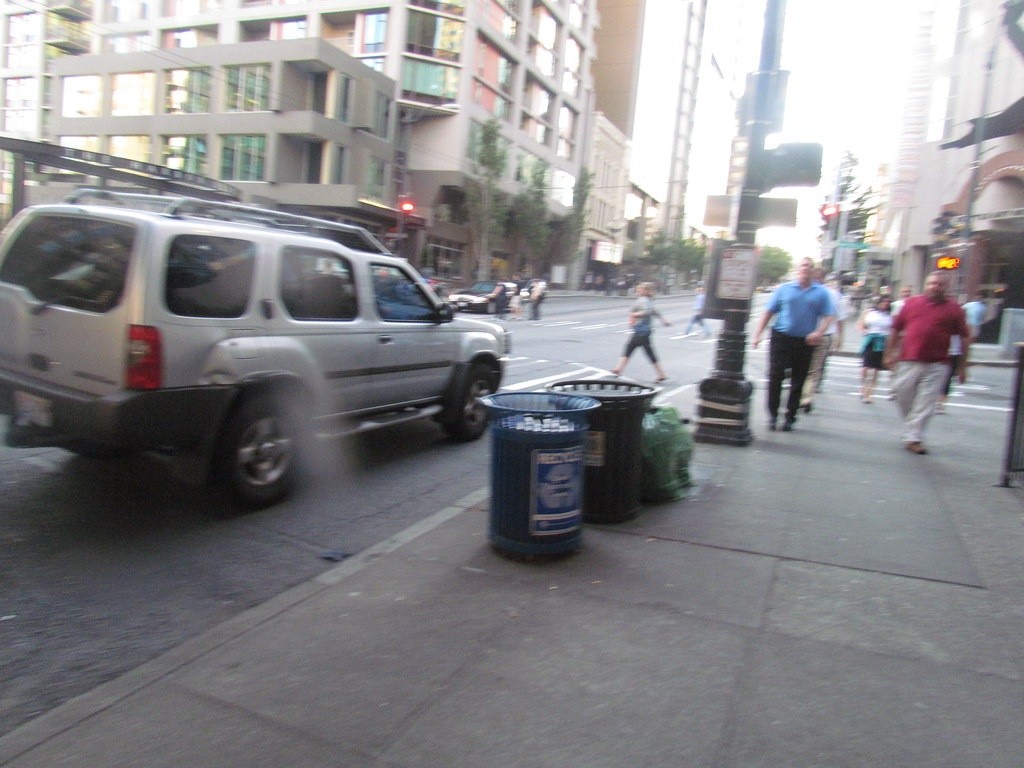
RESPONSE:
[448,279,520,313]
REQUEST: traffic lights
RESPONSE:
[818,201,839,232]
[398,200,415,219]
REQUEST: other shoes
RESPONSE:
[934,402,945,415]
[769,418,777,430]
[783,417,796,431]
[906,442,926,453]
[654,377,667,384]
[609,368,622,375]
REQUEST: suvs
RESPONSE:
[1,192,510,516]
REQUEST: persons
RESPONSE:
[683,287,711,337]
[751,256,988,457]
[490,267,674,322]
[607,282,672,383]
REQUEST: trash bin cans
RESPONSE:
[616,282,628,296]
[548,378,664,524]
[475,391,601,559]
